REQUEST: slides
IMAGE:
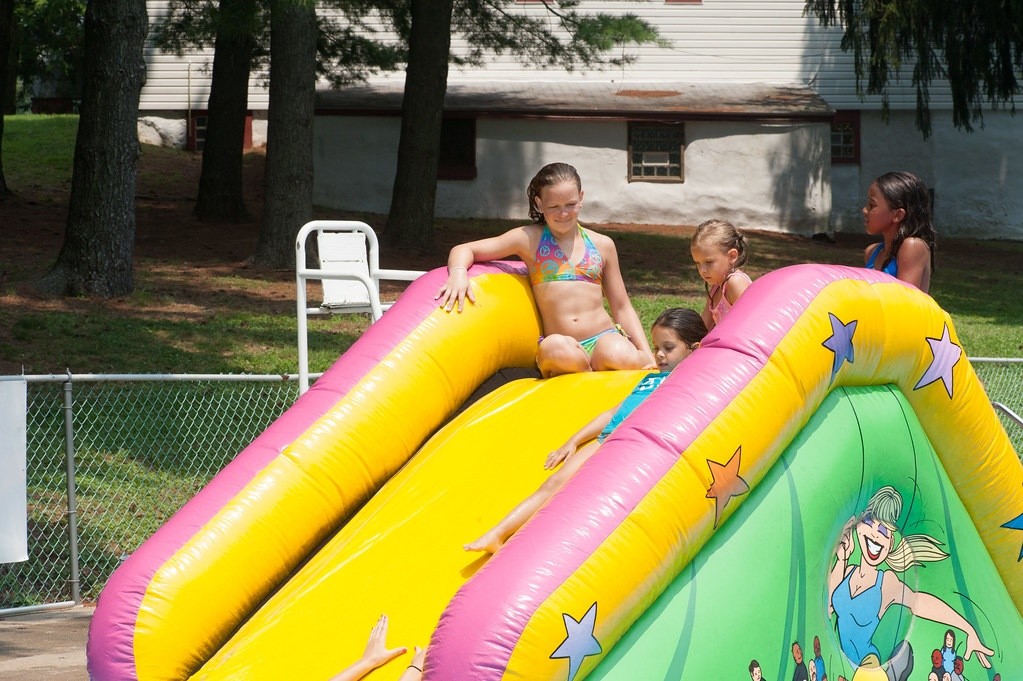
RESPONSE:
[79,249,1023,681]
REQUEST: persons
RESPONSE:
[649,219,754,367]
[329,612,428,681]
[464,310,709,555]
[863,170,937,299]
[434,163,656,376]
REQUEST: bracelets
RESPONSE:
[407,666,423,674]
